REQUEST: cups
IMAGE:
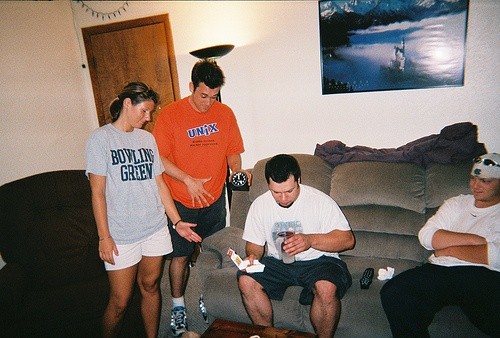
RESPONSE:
[275,231,296,264]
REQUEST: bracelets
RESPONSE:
[99,236,112,241]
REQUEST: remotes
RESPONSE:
[360,268,374,289]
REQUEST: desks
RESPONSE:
[201,316,320,337]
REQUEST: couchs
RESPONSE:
[182,152,486,338]
[0,170,149,338]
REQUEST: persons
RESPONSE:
[237,154,355,338]
[84,83,202,338]
[153,59,252,337]
[379,153,500,338]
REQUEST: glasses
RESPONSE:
[472,156,500,168]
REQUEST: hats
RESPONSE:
[471,152,500,179]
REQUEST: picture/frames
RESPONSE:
[318,0,469,95]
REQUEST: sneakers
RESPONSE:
[199,293,209,324]
[169,304,188,337]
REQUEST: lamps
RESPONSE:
[188,44,236,210]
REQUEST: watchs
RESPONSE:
[173,220,181,229]
